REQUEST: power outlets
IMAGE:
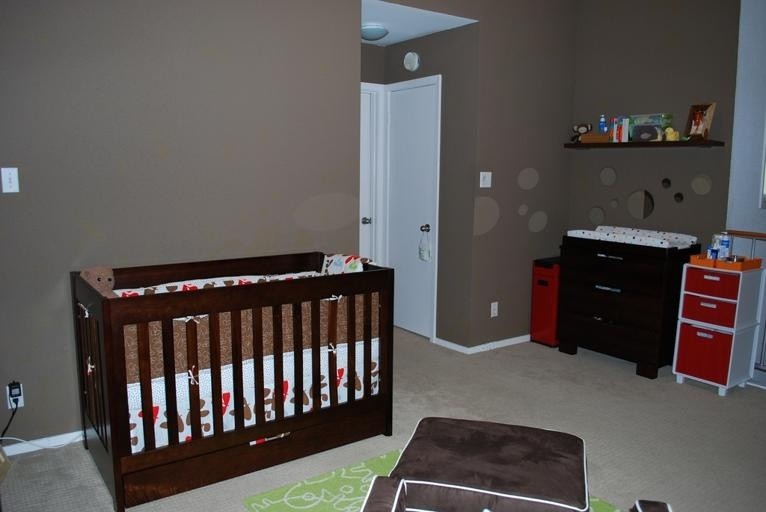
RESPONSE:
[1,167,20,193]
[6,383,24,409]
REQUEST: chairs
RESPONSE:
[358,476,672,512]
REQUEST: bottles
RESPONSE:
[598,114,607,130]
[721,230,729,258]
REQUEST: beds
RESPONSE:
[70,251,395,511]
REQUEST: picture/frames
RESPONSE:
[684,102,716,139]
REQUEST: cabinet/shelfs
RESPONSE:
[671,263,763,397]
[557,226,701,380]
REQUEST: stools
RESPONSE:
[386,416,590,512]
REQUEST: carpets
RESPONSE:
[241,447,626,512]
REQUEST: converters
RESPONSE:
[8,382,21,397]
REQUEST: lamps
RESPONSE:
[361,25,388,41]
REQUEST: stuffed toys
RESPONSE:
[568,121,593,144]
[79,261,119,303]
